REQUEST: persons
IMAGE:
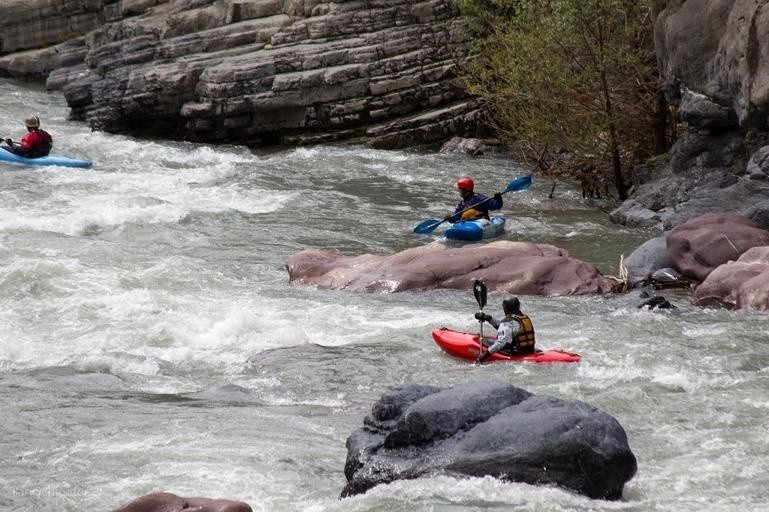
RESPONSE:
[0,112,53,160]
[475,295,536,364]
[443,175,505,223]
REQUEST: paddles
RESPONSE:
[472,280,487,353]
[0,137,21,145]
[414,174,531,234]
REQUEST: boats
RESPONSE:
[444,216,506,241]
[0,138,92,167]
[433,326,582,364]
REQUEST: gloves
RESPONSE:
[444,215,455,223]
[475,312,492,322]
[6,139,13,146]
[477,351,491,363]
[494,192,503,205]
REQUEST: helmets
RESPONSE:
[457,177,474,192]
[502,297,520,314]
[25,115,40,127]
